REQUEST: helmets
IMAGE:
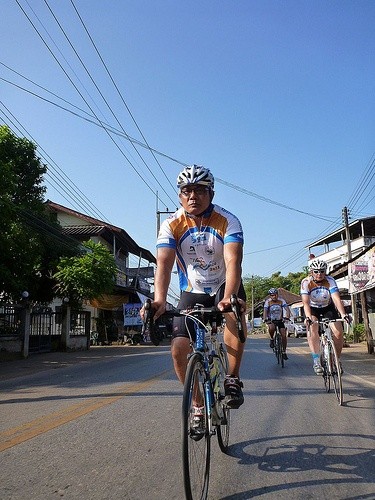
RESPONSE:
[308,259,327,270]
[268,288,278,295]
[176,164,214,190]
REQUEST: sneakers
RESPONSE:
[312,364,325,373]
[334,359,344,375]
[224,375,245,404]
[270,339,275,348]
[282,353,288,360]
[189,405,205,441]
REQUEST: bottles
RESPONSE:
[209,350,221,389]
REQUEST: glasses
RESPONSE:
[313,270,325,275]
[270,294,277,296]
[181,188,209,195]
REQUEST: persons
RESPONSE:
[263,289,290,360]
[139,165,246,442]
[300,260,353,376]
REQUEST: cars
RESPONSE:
[284,316,307,338]
[250,318,263,328]
[156,310,175,341]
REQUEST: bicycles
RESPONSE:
[140,298,246,500]
[265,318,291,367]
[305,314,352,406]
